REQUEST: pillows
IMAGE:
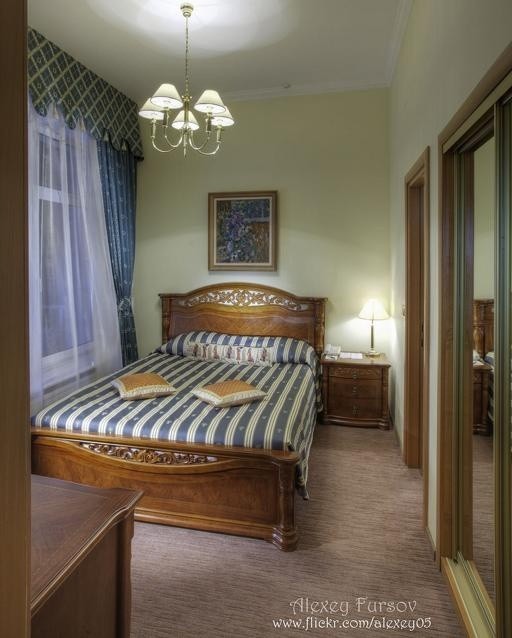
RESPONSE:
[155,330,315,363]
[110,371,178,399]
[191,377,267,407]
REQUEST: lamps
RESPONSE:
[137,0,234,157]
[360,298,390,356]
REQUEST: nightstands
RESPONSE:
[320,351,391,429]
[471,358,492,434]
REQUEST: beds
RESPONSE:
[29,281,329,548]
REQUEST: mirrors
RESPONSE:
[443,101,512,638]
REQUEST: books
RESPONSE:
[339,352,363,360]
[324,355,338,361]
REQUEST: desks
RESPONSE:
[30,468,142,635]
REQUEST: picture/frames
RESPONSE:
[207,189,279,269]
